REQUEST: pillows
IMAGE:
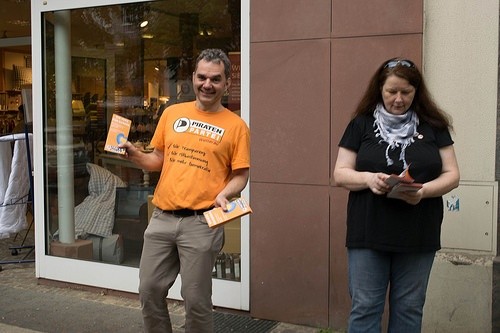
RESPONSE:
[12,64,32,90]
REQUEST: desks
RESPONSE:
[99,151,161,203]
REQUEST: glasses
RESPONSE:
[383,60,415,70]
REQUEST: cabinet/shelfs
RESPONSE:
[0,46,33,137]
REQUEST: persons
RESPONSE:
[118,48,251,333]
[333,57,460,333]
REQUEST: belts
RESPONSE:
[163,206,215,218]
